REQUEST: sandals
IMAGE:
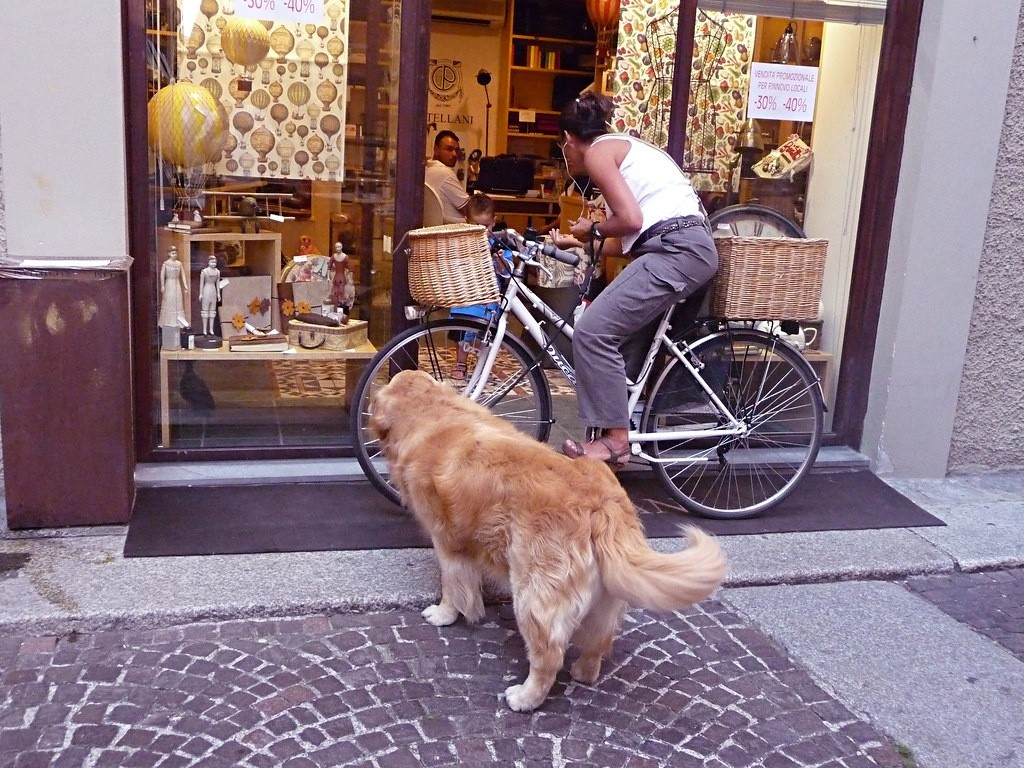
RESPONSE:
[450,362,468,380]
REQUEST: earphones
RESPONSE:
[563,141,567,147]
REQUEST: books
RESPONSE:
[511,44,560,69]
[229,334,288,352]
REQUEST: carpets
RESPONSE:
[122,470,947,564]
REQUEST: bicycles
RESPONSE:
[351,224,830,520]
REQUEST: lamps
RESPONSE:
[733,115,766,155]
[770,20,804,65]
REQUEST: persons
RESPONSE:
[158,245,188,329]
[549,90,719,474]
[328,242,350,306]
[198,255,221,335]
[425,130,470,219]
[447,194,514,379]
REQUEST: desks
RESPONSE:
[467,194,560,217]
[159,339,377,449]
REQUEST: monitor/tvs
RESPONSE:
[477,155,535,196]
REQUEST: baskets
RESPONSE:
[407,222,500,306]
[701,237,830,320]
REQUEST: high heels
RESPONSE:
[562,438,631,475]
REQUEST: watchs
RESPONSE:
[593,225,603,241]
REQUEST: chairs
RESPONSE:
[424,182,443,228]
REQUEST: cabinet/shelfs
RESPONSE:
[146,0,393,286]
[495,0,600,181]
[155,230,283,340]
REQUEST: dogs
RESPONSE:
[367,368,729,713]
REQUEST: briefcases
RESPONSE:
[288,316,368,351]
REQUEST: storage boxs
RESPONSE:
[288,320,369,348]
